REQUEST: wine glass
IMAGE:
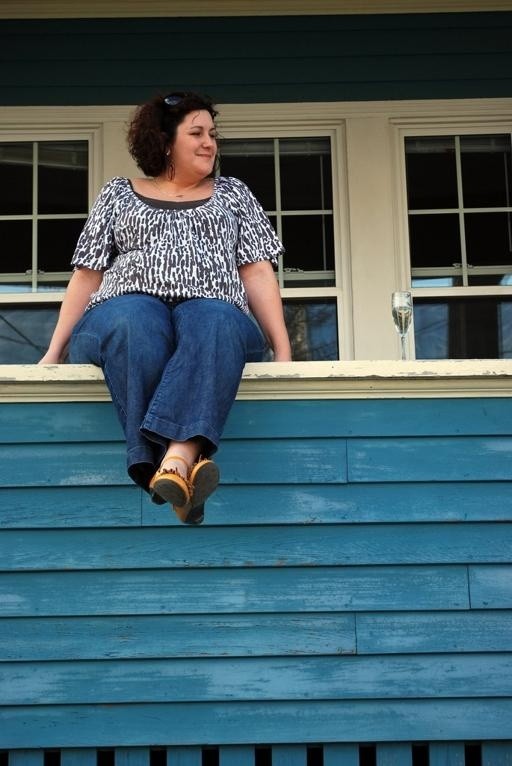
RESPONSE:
[389,288,414,358]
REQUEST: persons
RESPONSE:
[36,90,295,527]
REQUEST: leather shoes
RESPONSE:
[175,458,219,524]
[148,457,193,507]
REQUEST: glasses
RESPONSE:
[164,90,196,106]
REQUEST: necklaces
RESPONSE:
[148,178,202,198]
